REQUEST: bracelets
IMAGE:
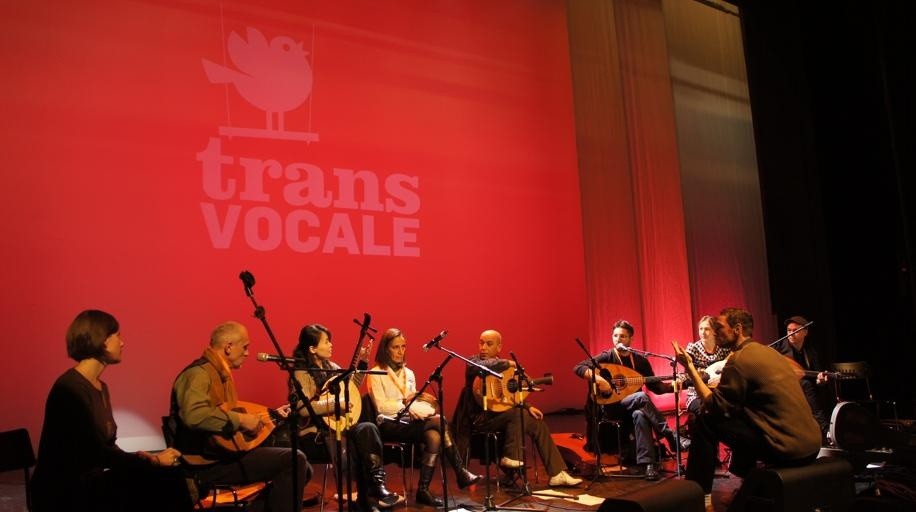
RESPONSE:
[153,454,162,465]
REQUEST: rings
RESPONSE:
[174,457,178,462]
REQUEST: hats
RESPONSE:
[786,317,809,326]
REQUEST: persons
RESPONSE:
[287,323,399,510]
[462,330,583,487]
[26,310,191,509]
[574,320,691,480]
[672,306,824,492]
[679,314,731,415]
[769,316,831,447]
[367,328,482,505]
[169,321,314,510]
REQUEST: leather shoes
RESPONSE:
[501,456,524,468]
[550,471,583,486]
[670,432,692,452]
[645,462,659,479]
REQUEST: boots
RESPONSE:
[443,431,482,488]
[354,452,406,512]
[416,448,443,505]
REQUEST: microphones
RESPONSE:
[257,352,306,367]
[616,343,646,359]
[505,377,544,394]
[422,329,447,352]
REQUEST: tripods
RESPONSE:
[495,351,579,508]
[457,371,542,512]
[655,362,730,484]
[571,366,646,491]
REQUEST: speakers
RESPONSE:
[596,480,705,512]
[725,458,856,512]
